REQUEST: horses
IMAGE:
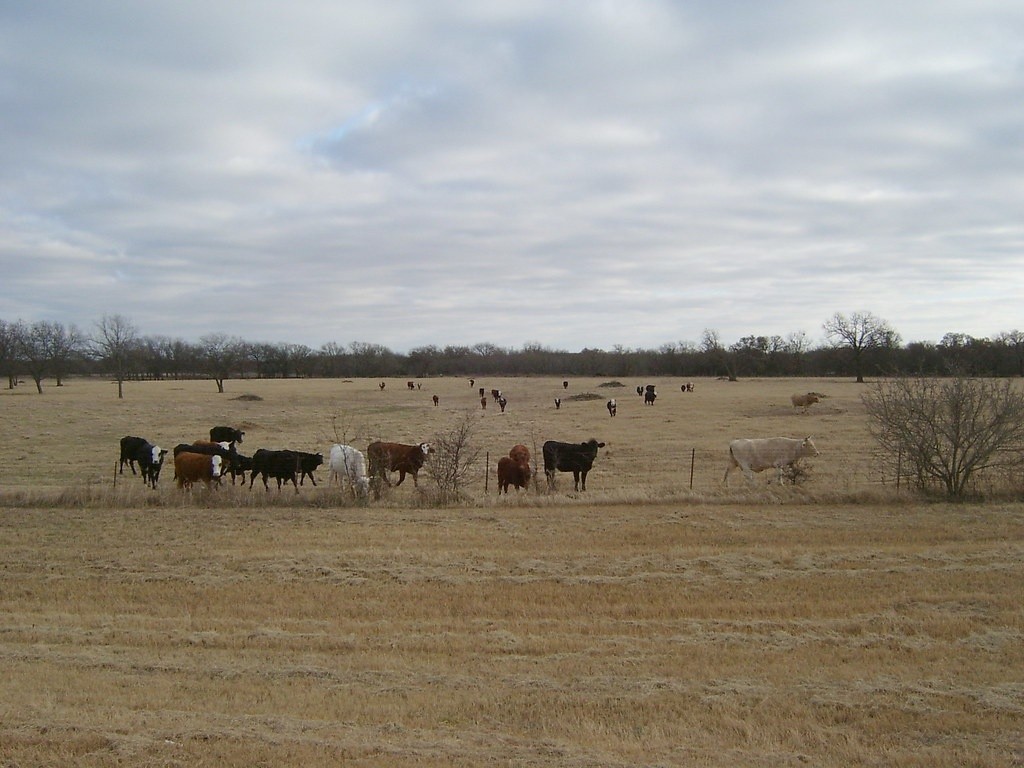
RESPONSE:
[721,435,820,490]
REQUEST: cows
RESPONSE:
[172,425,324,497]
[636,384,659,406]
[367,441,437,489]
[119,436,168,491]
[607,399,617,418]
[433,395,439,407]
[790,391,821,415]
[328,444,373,495]
[681,382,694,393]
[479,387,487,410]
[554,398,561,409]
[563,381,569,389]
[543,438,606,493]
[497,445,533,496]
[378,381,385,391]
[468,379,475,388]
[417,382,422,390]
[491,389,507,412]
[408,381,416,390]
